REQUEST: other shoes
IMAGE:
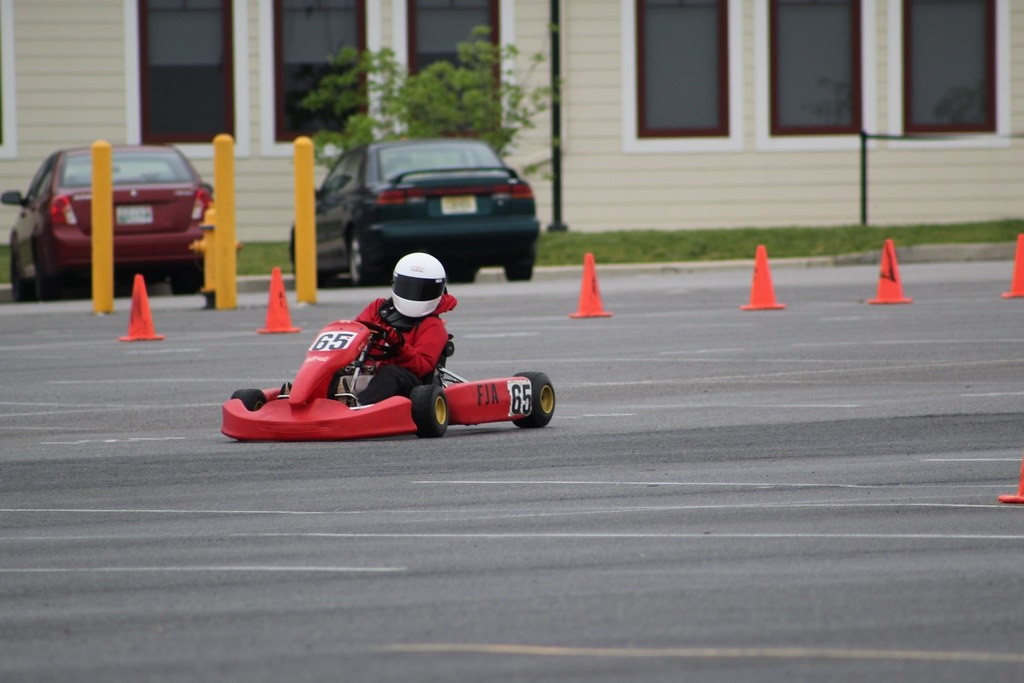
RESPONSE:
[280,382,292,398]
[333,377,353,407]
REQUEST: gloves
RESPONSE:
[381,323,401,347]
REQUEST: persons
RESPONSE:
[328,253,457,408]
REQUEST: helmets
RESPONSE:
[392,253,446,317]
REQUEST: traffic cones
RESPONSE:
[996,452,1024,504]
[999,232,1024,298]
[117,274,164,343]
[256,266,301,333]
[568,253,615,317]
[865,237,913,305]
[739,245,787,310]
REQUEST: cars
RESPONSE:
[288,136,540,289]
[0,142,213,304]
